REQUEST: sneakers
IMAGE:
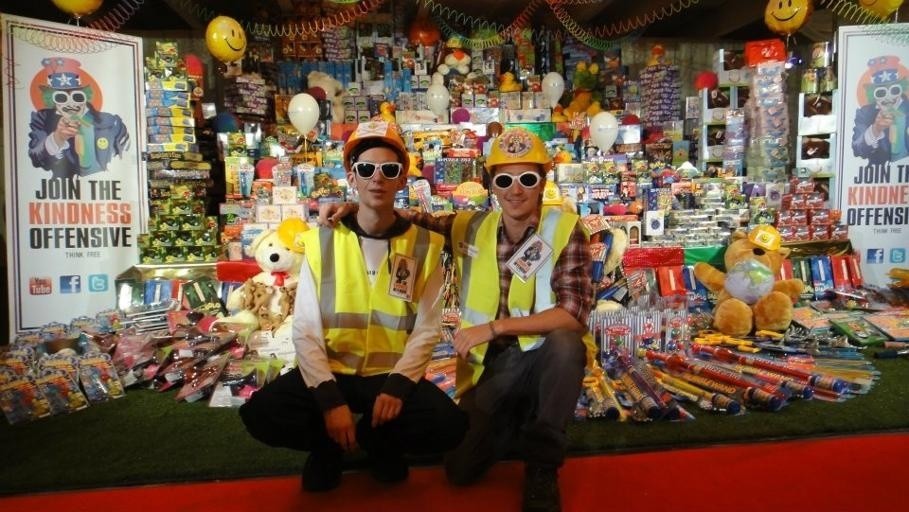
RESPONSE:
[372,452,410,486]
[301,455,341,495]
[522,465,561,512]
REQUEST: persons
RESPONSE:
[29,72,131,177]
[243,121,465,491]
[852,69,909,165]
[317,127,592,512]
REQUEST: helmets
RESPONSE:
[343,119,411,174]
[484,127,552,171]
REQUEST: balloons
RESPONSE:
[857,0,902,16]
[761,0,809,33]
[288,93,320,136]
[540,73,566,109]
[588,112,619,152]
[203,14,247,61]
[52,0,102,18]
[426,85,450,117]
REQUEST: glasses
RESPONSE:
[873,85,901,101]
[351,161,403,180]
[53,90,86,104]
[492,173,542,189]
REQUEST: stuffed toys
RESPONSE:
[228,217,309,330]
[694,221,803,335]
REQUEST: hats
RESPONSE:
[864,57,906,86]
[39,55,90,92]
[747,223,783,252]
[277,217,313,255]
